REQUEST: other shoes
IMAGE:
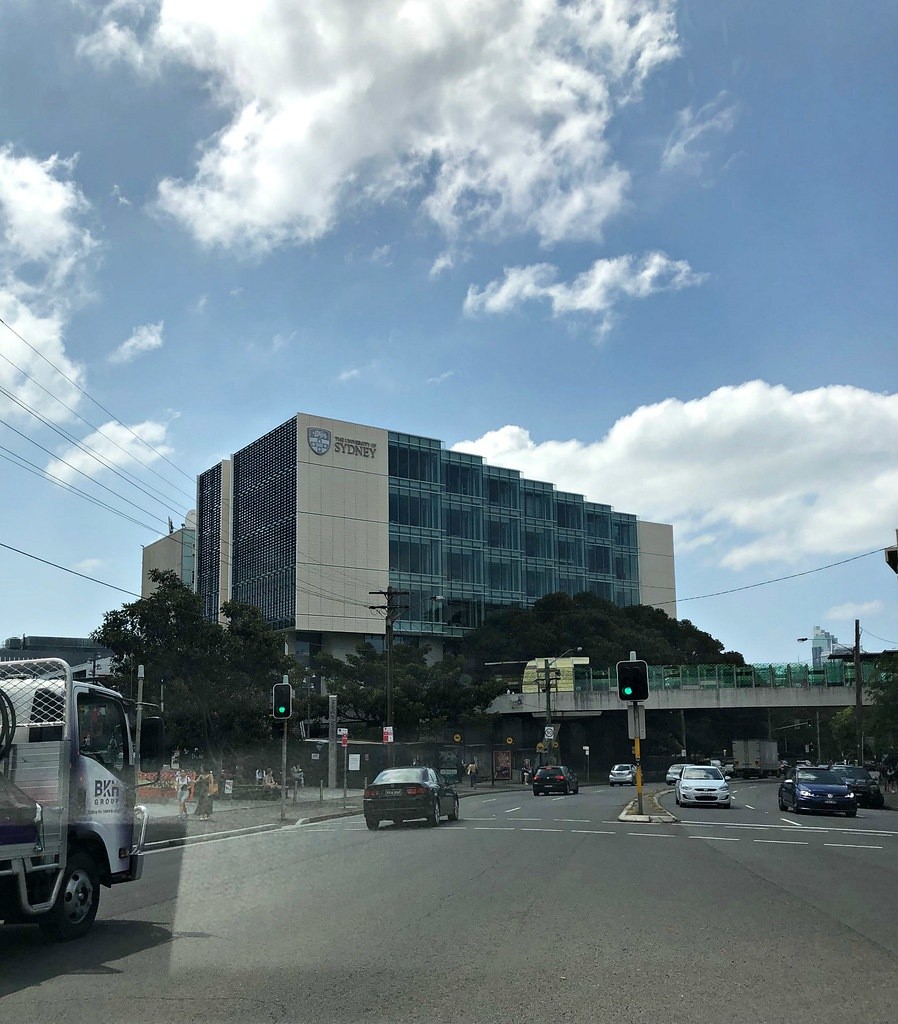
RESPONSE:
[474,787,476,789]
[179,814,188,818]
[199,817,211,821]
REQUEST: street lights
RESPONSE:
[545,646,585,765]
[796,620,864,765]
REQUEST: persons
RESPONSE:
[188,764,219,820]
[523,762,532,786]
[256,769,266,786]
[881,764,898,794]
[467,760,478,786]
[289,764,305,788]
[262,768,277,788]
[174,769,191,818]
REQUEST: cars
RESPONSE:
[826,764,884,809]
[674,765,731,809]
[608,763,644,786]
[362,765,460,830]
[777,764,859,817]
[665,764,695,785]
[699,759,737,779]
[531,765,579,796]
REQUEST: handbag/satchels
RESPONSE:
[467,769,470,775]
[475,769,479,775]
[208,784,218,797]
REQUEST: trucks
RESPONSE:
[0,654,149,938]
[732,738,781,779]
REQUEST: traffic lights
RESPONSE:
[272,683,294,720]
[617,660,650,702]
